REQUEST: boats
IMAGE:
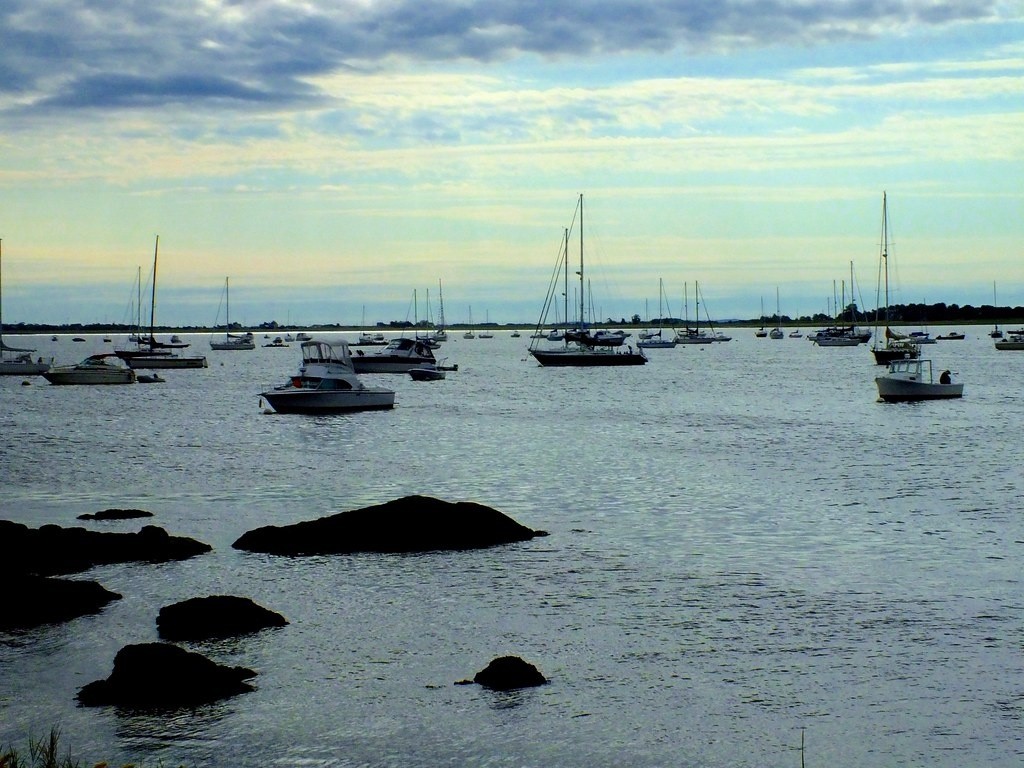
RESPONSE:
[374,333,384,340]
[995,329,1024,350]
[872,358,965,403]
[257,338,395,410]
[408,364,446,380]
[907,332,936,345]
[40,355,135,385]
[136,374,165,382]
[708,333,732,341]
[346,338,436,371]
[510,330,520,337]
[169,335,182,343]
[53,337,57,342]
[72,338,86,342]
[262,333,312,347]
[530,332,548,338]
[104,338,111,342]
[935,332,966,340]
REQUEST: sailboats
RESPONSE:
[807,261,873,347]
[567,279,632,345]
[0,238,46,373]
[402,277,448,349]
[463,305,475,339]
[755,295,768,338]
[637,276,718,348]
[545,293,564,342]
[871,189,922,366]
[114,234,206,368]
[527,193,647,366]
[989,280,1003,338]
[208,275,256,350]
[357,305,374,342]
[788,310,803,338]
[770,286,784,339]
[479,309,493,338]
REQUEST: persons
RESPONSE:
[939,370,951,384]
